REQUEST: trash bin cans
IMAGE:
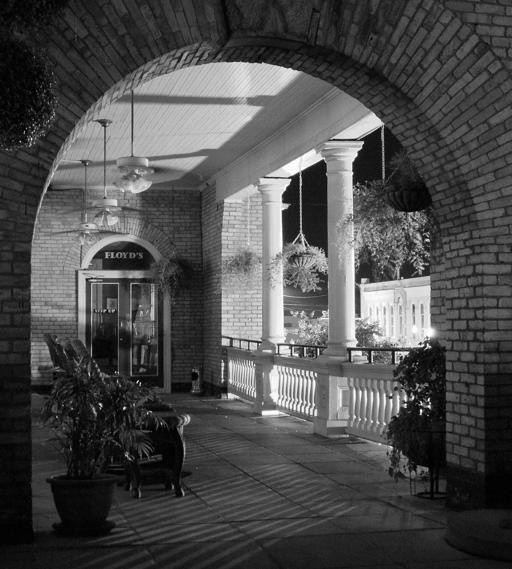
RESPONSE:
[191,368,202,394]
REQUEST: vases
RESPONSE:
[287,253,317,268]
[380,183,429,213]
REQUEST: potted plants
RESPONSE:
[384,336,446,483]
[271,241,329,294]
[36,353,169,537]
[335,150,430,278]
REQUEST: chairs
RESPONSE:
[43,332,193,498]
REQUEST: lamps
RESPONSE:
[75,90,153,247]
